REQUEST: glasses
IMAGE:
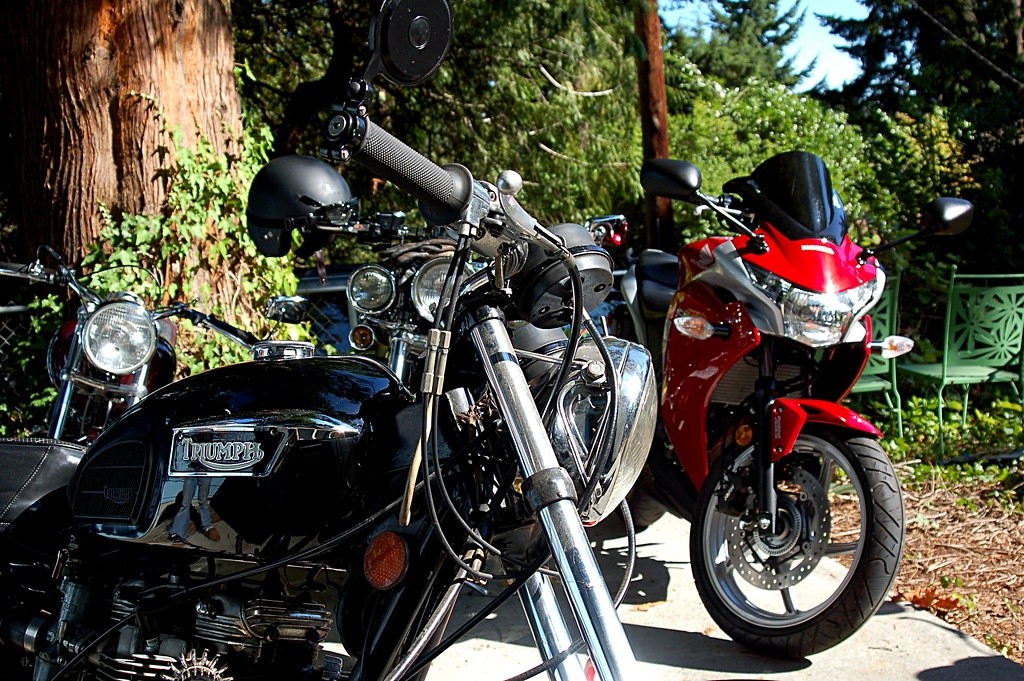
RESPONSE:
[321,196,359,226]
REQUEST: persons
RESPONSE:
[170,432,225,541]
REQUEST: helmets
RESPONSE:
[245,155,357,258]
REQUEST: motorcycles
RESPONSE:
[0,1,685,680]
[590,150,976,657]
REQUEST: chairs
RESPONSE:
[896,264,1024,461]
[815,265,905,460]
